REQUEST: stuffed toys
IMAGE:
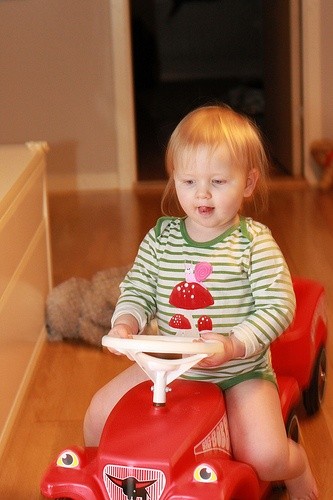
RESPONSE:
[46,266,132,349]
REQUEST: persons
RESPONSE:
[83,105,320,500]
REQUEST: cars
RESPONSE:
[39,274,326,500]
